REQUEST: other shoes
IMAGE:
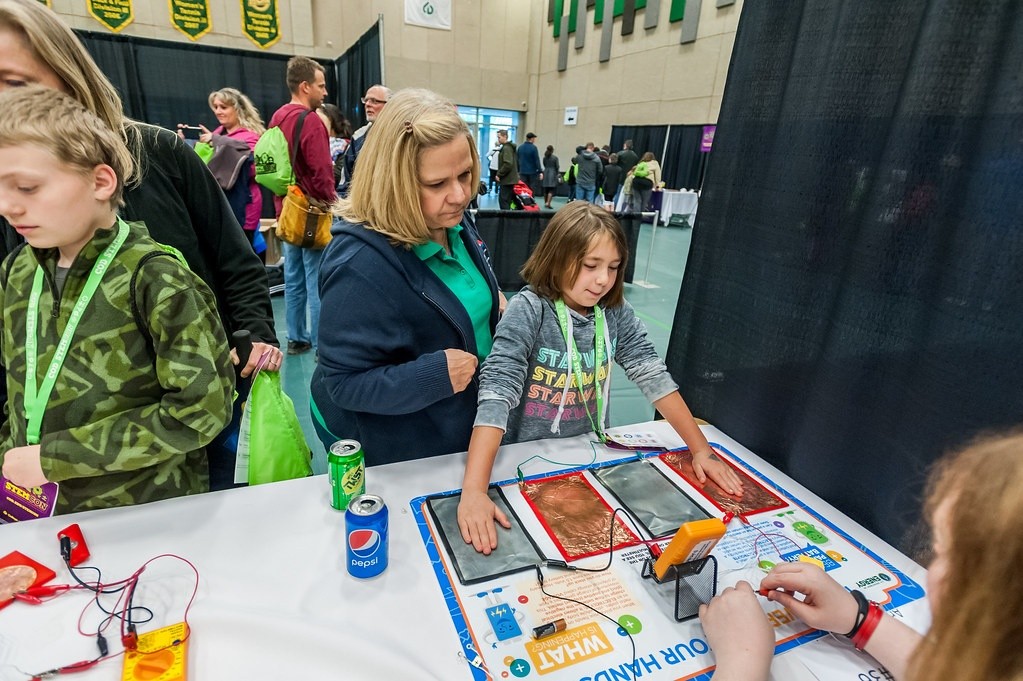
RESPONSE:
[287,340,315,354]
[567,199,571,203]
[545,204,553,209]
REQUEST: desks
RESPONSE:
[0,418,932,681]
[615,185,698,227]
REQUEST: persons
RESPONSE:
[0,0,283,493]
[0,86,236,524]
[697,431,1023,681]
[309,87,507,468]
[564,139,662,213]
[485,127,559,209]
[177,55,396,363]
[457,200,744,555]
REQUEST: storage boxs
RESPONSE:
[256,215,282,264]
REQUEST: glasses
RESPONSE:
[361,97,387,106]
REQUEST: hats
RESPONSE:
[526,132,537,138]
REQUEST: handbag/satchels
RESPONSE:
[193,140,215,165]
[633,176,653,192]
[276,183,333,251]
[510,179,540,212]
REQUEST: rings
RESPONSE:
[269,361,277,366]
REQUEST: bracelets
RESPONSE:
[847,584,883,652]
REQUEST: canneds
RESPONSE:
[344,494,388,579]
[327,438,365,511]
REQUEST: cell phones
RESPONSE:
[179,127,203,140]
[57,524,91,568]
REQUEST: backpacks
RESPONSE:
[254,106,309,196]
